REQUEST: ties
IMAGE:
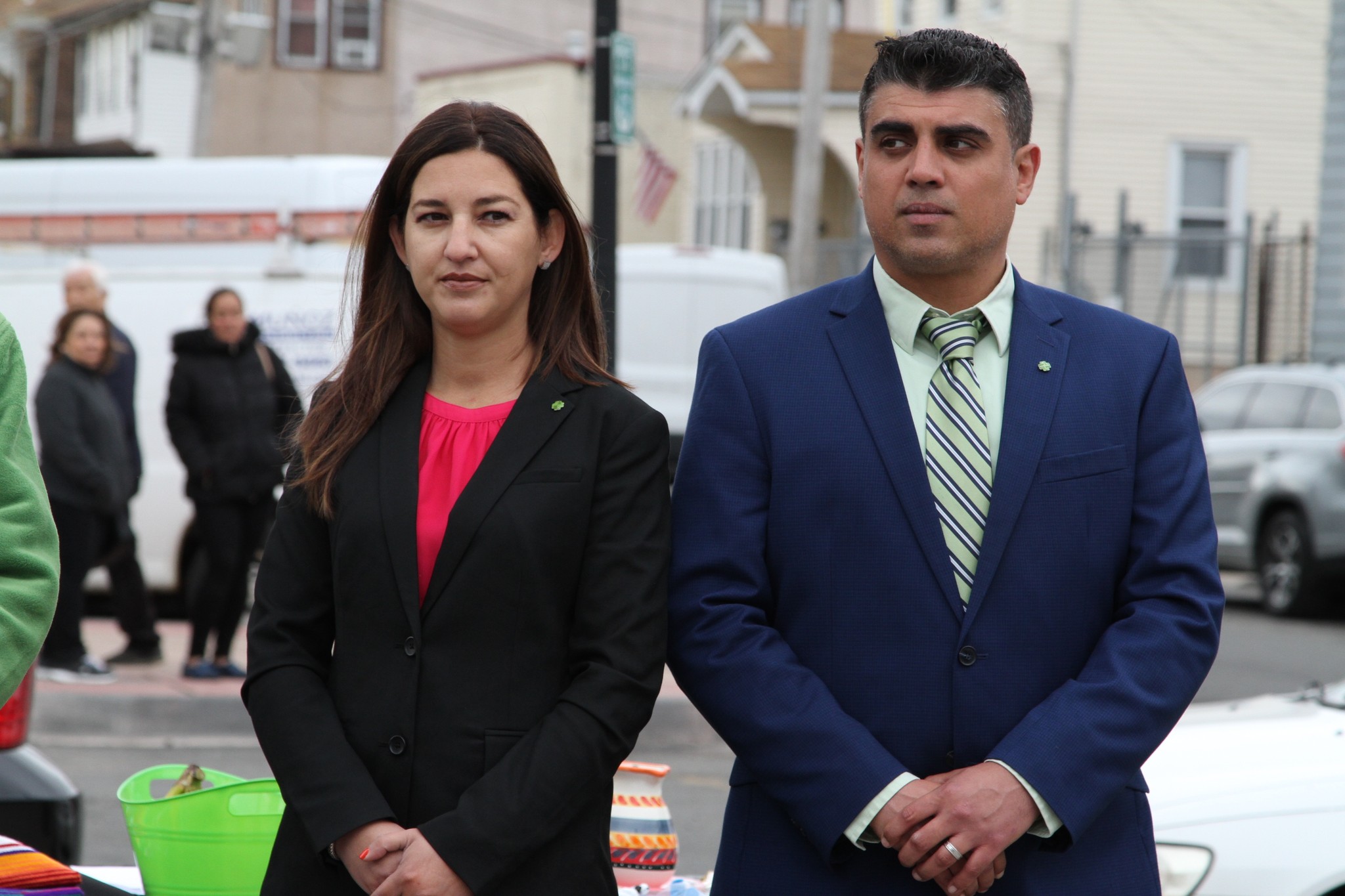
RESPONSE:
[916,314,995,612]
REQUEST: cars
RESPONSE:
[0,666,86,868]
[1138,676,1345,896]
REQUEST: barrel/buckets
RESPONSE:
[117,763,286,895]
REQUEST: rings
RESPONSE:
[943,840,963,861]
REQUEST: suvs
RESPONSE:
[1192,360,1344,621]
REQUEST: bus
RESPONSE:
[1,150,395,595]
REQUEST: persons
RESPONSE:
[668,27,1226,896]
[164,286,306,680]
[237,101,669,895]
[0,258,162,711]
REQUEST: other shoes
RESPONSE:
[185,662,218,680]
[36,656,115,685]
[103,645,161,665]
[210,664,247,677]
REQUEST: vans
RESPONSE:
[617,245,783,435]
